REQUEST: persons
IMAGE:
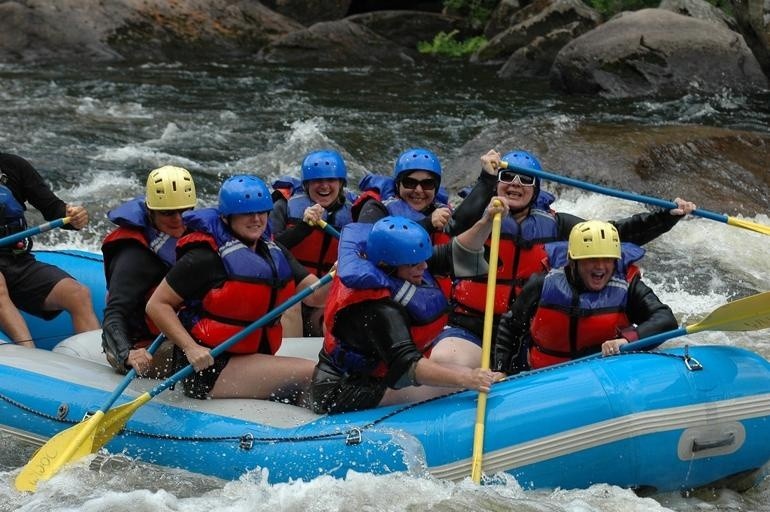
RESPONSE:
[99,147,698,415]
[1,151,100,350]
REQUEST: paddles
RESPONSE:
[28,266,334,482]
[16,332,165,493]
[619,289,770,349]
[495,160,769,235]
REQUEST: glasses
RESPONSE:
[402,176,437,190]
[496,169,537,187]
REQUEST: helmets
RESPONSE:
[392,149,442,194]
[365,215,434,268]
[145,164,197,210]
[567,220,622,261]
[302,149,348,191]
[219,175,275,217]
[499,151,542,183]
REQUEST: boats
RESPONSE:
[0,248,769,503]
